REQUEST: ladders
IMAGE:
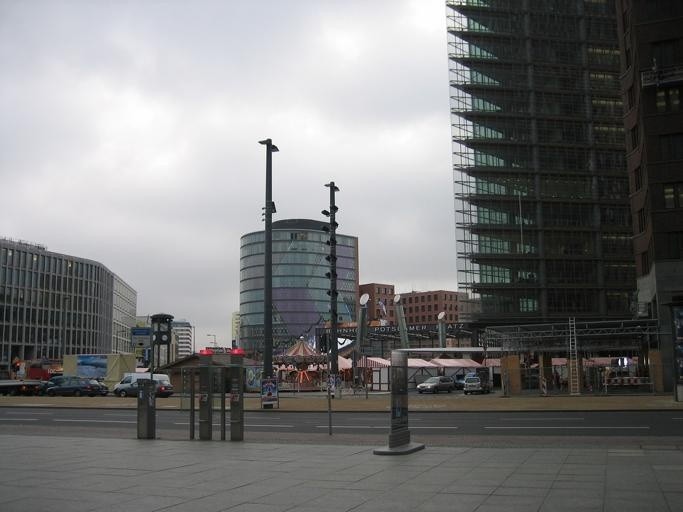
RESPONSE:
[569,317,580,396]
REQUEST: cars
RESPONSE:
[454,374,490,395]
[416,376,455,394]
[0,372,175,397]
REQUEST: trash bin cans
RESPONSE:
[674,379,683,402]
